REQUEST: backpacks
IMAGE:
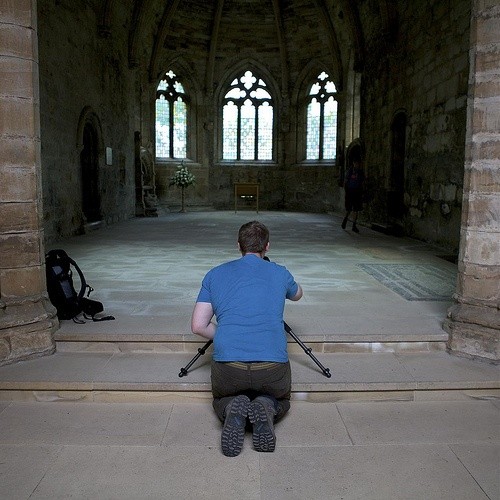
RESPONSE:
[44,248,116,324]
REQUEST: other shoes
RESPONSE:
[248,397,276,452]
[221,395,250,458]
[352,226,359,233]
[341,220,348,229]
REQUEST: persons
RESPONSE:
[191,220,303,455]
[341,138,364,232]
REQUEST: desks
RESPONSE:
[233,182,259,215]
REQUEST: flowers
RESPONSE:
[168,159,200,190]
